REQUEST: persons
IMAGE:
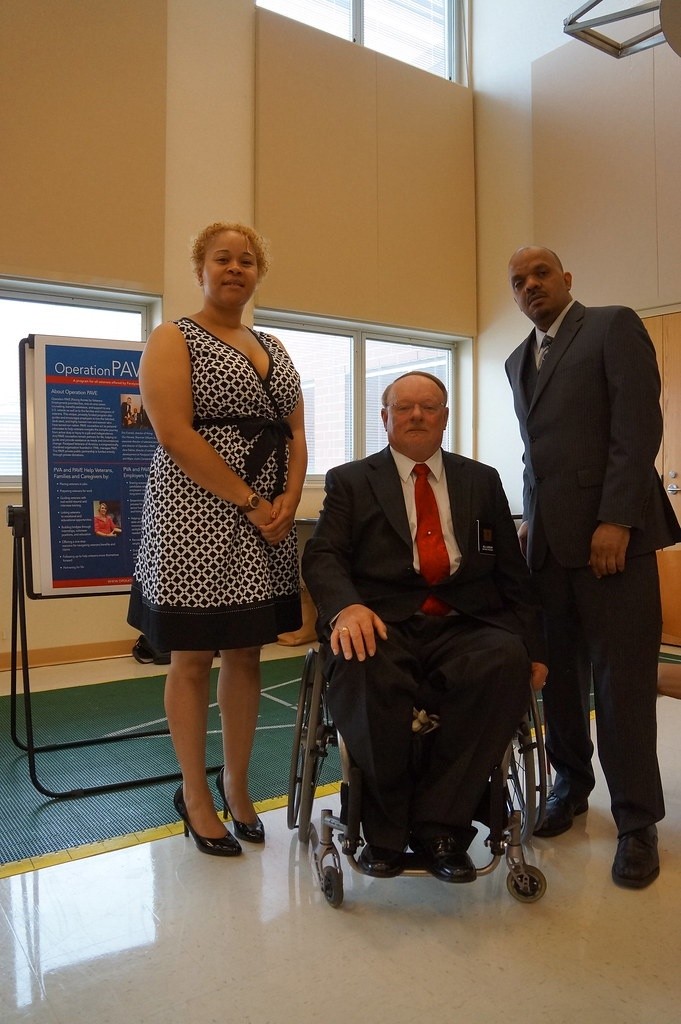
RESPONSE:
[505,246,681,890]
[303,372,550,883]
[122,397,148,428]
[94,503,122,537]
[127,223,307,857]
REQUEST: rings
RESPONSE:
[340,627,347,632]
[543,682,546,685]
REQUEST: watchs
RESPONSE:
[240,490,260,513]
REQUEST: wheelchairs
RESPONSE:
[286,642,552,909]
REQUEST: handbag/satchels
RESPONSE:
[131,632,221,665]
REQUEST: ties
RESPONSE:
[412,463,452,617]
[537,335,552,375]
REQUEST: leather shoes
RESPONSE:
[610,822,660,889]
[532,790,589,838]
[414,830,477,883]
[358,844,407,879]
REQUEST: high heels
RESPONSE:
[215,766,265,844]
[173,783,242,857]
